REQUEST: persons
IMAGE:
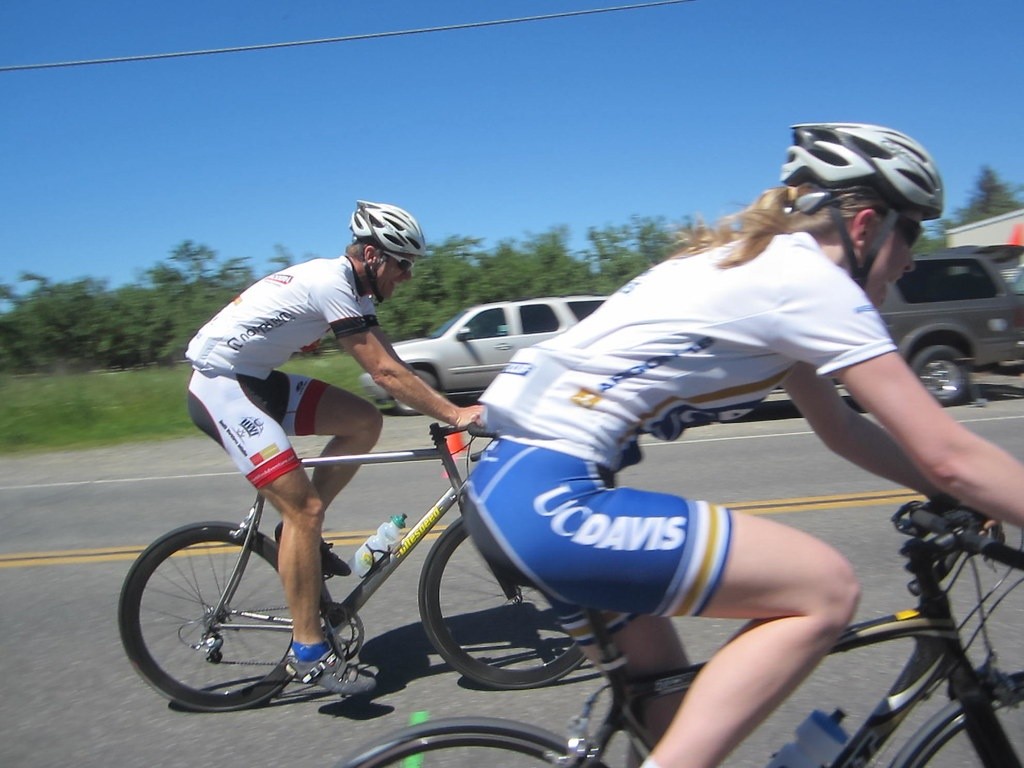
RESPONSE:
[186,201,486,694]
[464,123,1024,768]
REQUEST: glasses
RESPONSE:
[868,195,925,247]
[367,239,417,274]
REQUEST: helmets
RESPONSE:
[349,200,426,259]
[779,123,945,224]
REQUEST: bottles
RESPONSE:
[765,708,851,768]
[347,513,408,579]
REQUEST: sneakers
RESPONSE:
[274,522,353,576]
[285,645,385,694]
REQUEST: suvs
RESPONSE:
[784,243,1024,406]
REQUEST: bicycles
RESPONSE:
[318,482,1024,768]
[116,393,594,713]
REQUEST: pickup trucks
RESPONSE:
[358,295,614,417]
